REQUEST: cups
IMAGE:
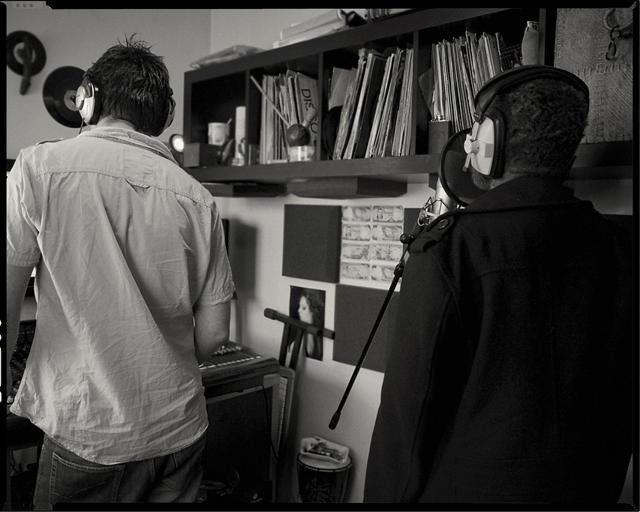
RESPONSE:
[208,123,229,146]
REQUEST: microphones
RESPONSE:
[428,129,471,226]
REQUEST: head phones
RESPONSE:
[75,68,175,135]
[465,67,588,180]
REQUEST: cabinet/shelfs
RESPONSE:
[181,8,633,181]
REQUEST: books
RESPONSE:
[260,71,319,164]
[332,21,539,160]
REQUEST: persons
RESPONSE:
[6,45,234,512]
[290,292,324,361]
[366,77,633,506]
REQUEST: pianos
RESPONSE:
[3,320,278,430]
